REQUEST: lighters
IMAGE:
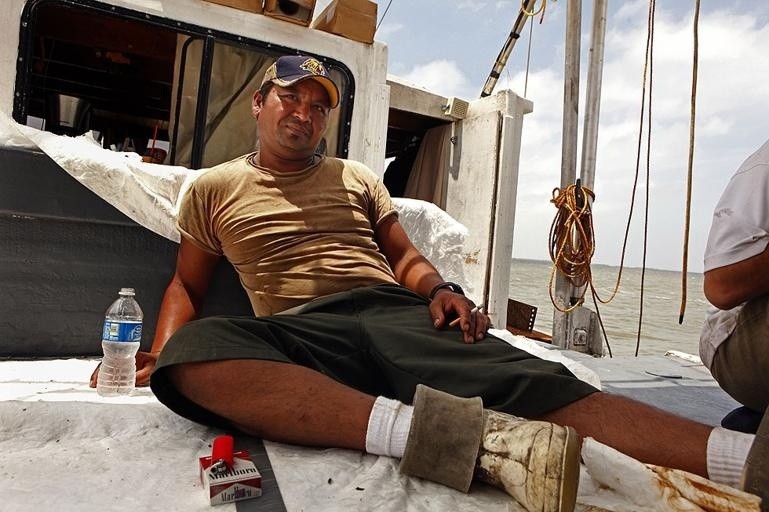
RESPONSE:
[210,435,234,474]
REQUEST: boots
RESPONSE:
[397,383,583,512]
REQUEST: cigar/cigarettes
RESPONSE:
[448,303,484,326]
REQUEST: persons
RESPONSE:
[700,139,769,432]
[89,53,769,511]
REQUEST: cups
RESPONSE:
[58,93,80,130]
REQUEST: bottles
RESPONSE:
[95,286,146,396]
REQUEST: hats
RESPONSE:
[261,56,339,109]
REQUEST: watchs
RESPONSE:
[427,282,465,300]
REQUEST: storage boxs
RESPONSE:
[264,0,319,25]
[311,0,381,48]
[202,0,267,14]
